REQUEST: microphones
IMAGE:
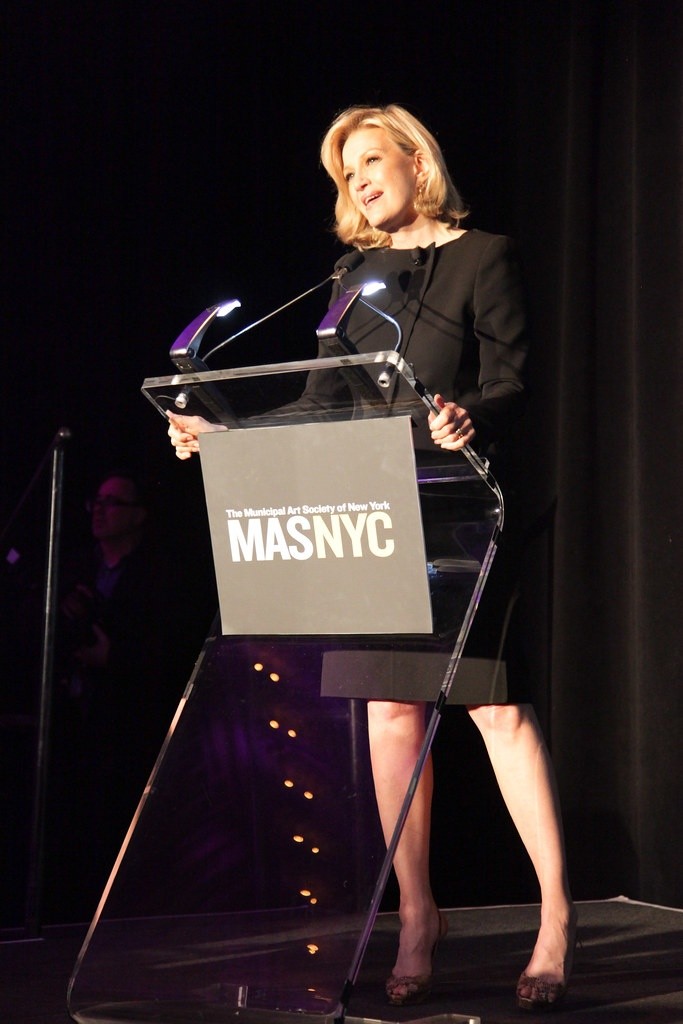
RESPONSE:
[202,250,403,363]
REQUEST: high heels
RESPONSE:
[515,910,580,1015]
[384,910,449,1007]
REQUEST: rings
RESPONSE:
[456,429,464,441]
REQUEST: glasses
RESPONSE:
[86,494,144,516]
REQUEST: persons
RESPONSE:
[44,472,191,933]
[166,104,578,1012]
